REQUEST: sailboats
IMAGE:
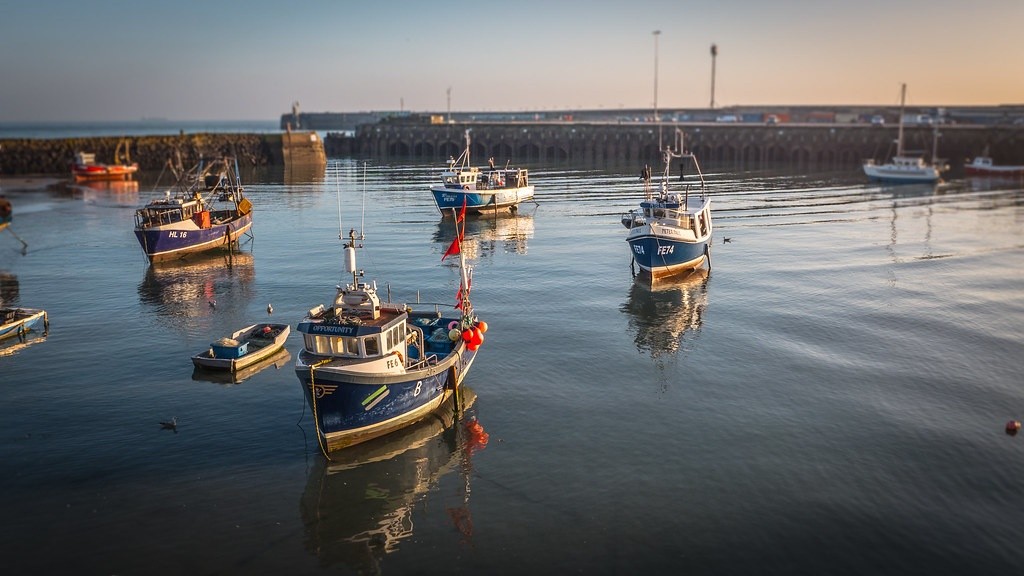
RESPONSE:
[862,79,942,185]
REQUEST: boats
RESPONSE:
[191,347,290,385]
[621,139,713,281]
[431,208,534,270]
[133,143,255,264]
[137,245,254,329]
[428,130,541,216]
[961,140,1024,175]
[619,265,718,391]
[190,321,290,374]
[0,306,49,340]
[0,201,13,230]
[295,152,488,451]
[49,177,139,209]
[0,331,48,356]
[70,134,138,181]
[297,381,488,575]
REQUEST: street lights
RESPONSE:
[651,28,661,121]
[710,44,717,110]
